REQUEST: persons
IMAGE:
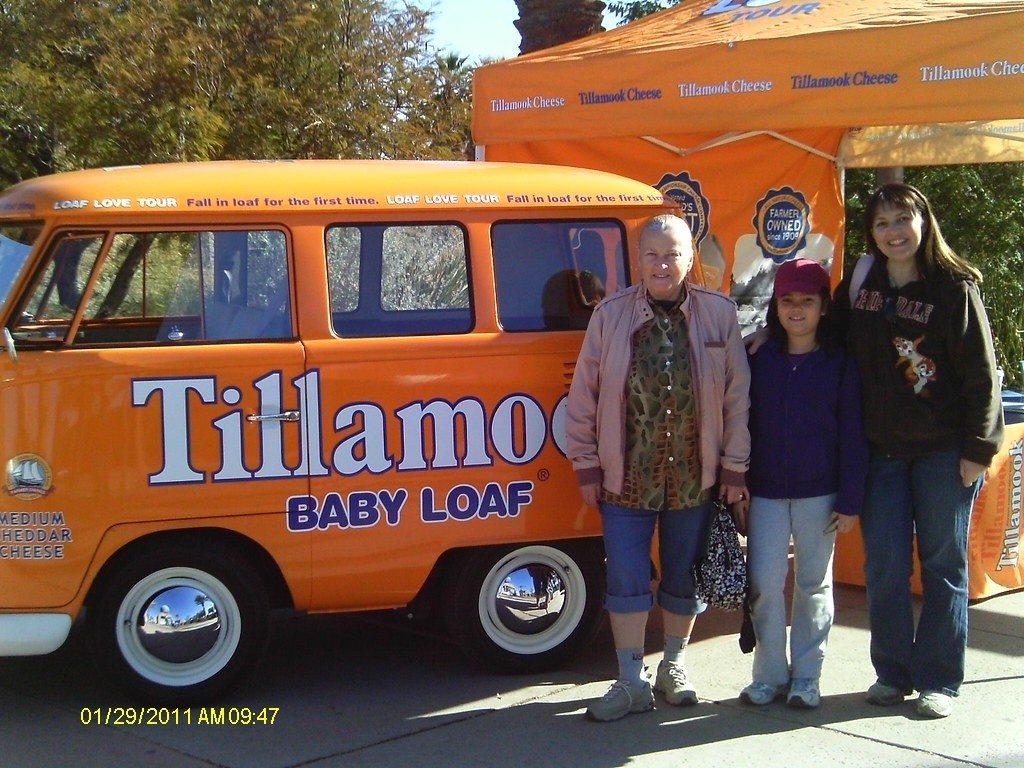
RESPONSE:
[732,258,868,707]
[165,615,169,626]
[543,569,555,614]
[565,214,752,721]
[541,268,606,330]
[519,586,523,597]
[742,182,1006,718]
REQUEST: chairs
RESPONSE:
[226,301,286,342]
[197,300,243,342]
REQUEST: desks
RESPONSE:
[831,410,1024,603]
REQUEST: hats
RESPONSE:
[773,257,831,298]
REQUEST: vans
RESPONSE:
[0,158,706,712]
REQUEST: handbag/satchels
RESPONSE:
[690,495,748,612]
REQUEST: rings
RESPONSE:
[740,495,743,498]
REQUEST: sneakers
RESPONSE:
[784,677,821,708]
[653,659,698,706]
[916,689,953,717]
[738,681,790,706]
[585,677,655,722]
[865,681,904,705]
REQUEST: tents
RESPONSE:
[472,0,1024,600]
